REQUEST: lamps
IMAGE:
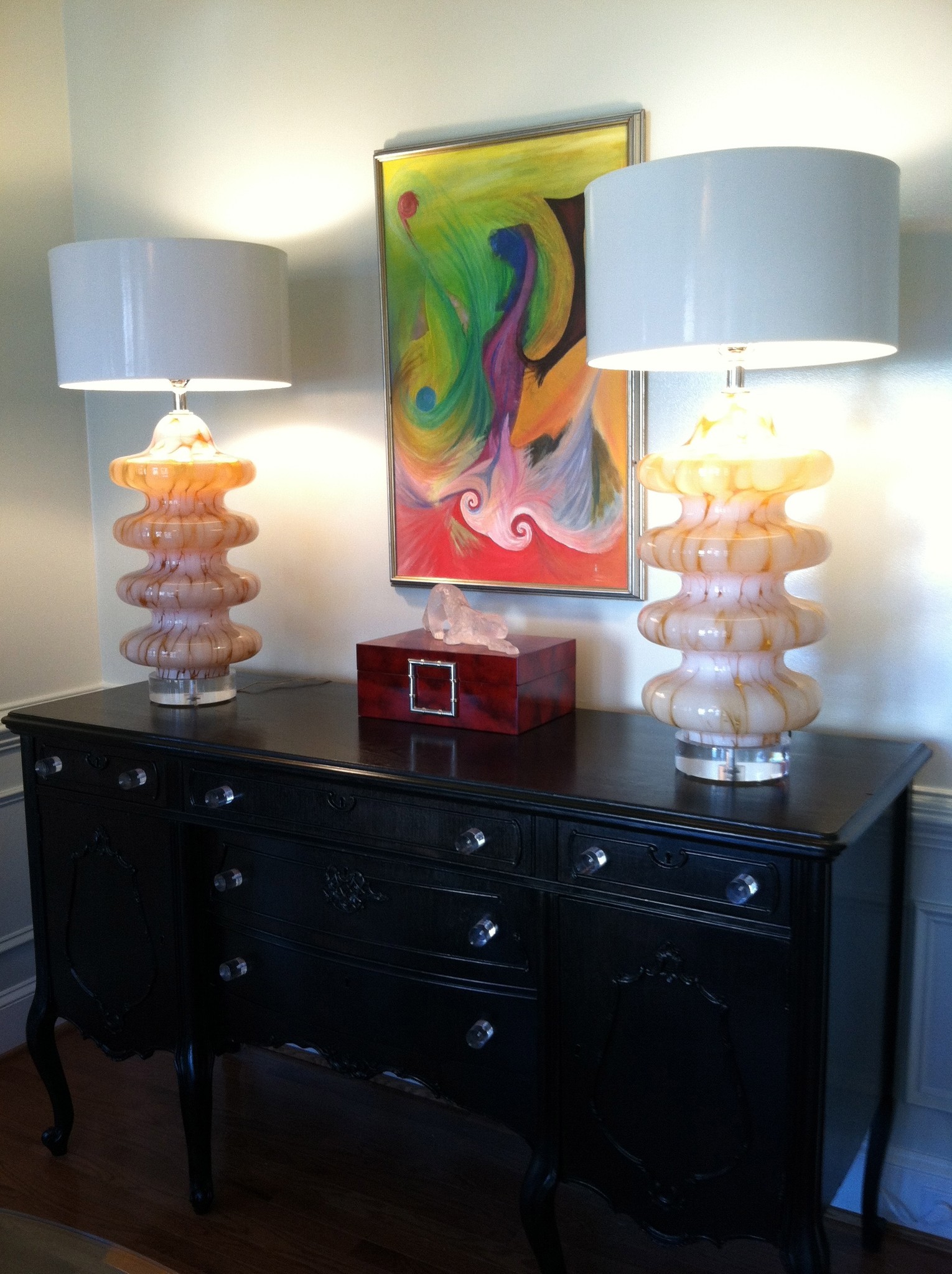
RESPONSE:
[580,145,904,786]
[46,239,292,708]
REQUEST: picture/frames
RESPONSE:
[374,109,653,604]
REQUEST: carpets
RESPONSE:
[0,1209,178,1274]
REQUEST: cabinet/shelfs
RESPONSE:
[0,664,931,1274]
